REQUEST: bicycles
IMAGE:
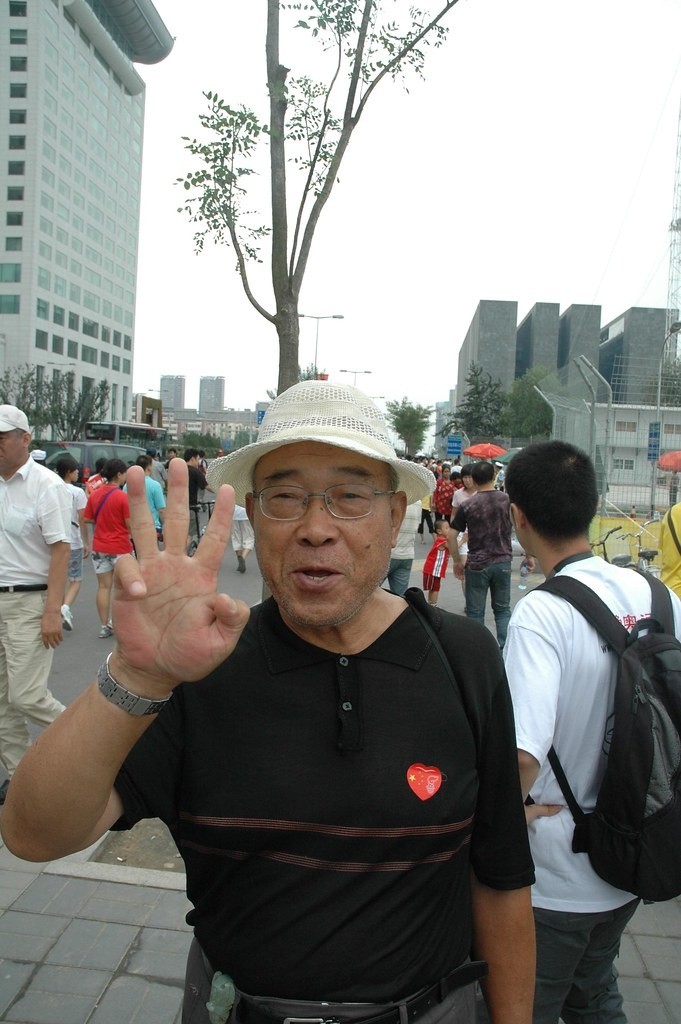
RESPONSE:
[616,519,661,579]
[588,526,654,577]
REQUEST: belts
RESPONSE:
[0,584,47,592]
[237,960,488,1024]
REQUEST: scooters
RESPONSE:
[186,499,216,559]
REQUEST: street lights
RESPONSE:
[339,369,372,388]
[648,316,681,522]
[298,313,345,379]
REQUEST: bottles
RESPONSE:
[201,526,206,536]
[518,563,528,589]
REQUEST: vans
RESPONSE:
[37,441,146,493]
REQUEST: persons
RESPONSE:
[383,454,504,544]
[0,380,537,1024]
[422,519,450,604]
[84,448,215,638]
[30,441,47,466]
[450,463,477,597]
[657,501,681,597]
[56,452,90,631]
[231,504,254,573]
[379,500,422,595]
[446,462,536,651]
[217,450,224,458]
[0,404,73,803]
[502,440,681,1024]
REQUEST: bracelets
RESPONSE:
[98,653,173,716]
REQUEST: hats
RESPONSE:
[0,404,30,432]
[29,449,46,460]
[496,462,504,468]
[146,448,156,455]
[204,380,437,505]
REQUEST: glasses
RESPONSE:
[253,483,397,519]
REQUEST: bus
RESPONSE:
[84,421,172,462]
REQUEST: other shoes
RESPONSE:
[60,606,74,631]
[238,555,246,574]
[99,627,113,638]
[0,779,10,805]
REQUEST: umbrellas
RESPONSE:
[465,442,508,460]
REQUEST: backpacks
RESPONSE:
[526,570,681,905]
